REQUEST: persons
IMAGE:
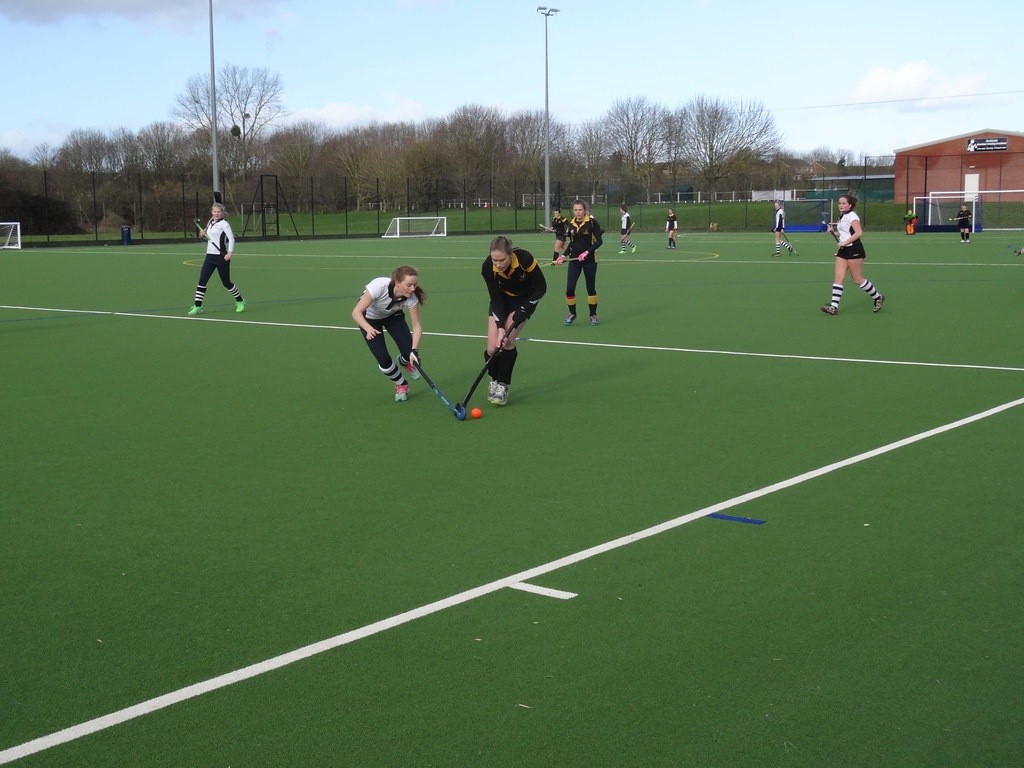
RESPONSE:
[618,206,636,254]
[545,210,570,261]
[665,208,678,249]
[352,266,427,402]
[820,195,885,315]
[773,201,793,257]
[904,210,919,235]
[481,237,547,404]
[556,200,604,325]
[954,204,973,242]
[188,203,248,314]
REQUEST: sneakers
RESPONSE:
[820,304,838,315]
[486,379,507,406]
[188,304,207,315]
[236,299,246,312]
[873,294,885,312]
[564,312,576,325]
[395,354,420,380]
[591,315,597,325]
[395,384,408,401]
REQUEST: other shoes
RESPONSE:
[631,246,636,254]
[772,253,780,257]
[619,251,627,254]
[788,247,793,256]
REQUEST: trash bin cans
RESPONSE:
[121,225,132,245]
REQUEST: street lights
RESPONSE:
[535,5,560,231]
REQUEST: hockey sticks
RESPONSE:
[413,361,466,421]
[782,232,799,256]
[821,212,844,249]
[539,223,564,235]
[949,216,968,220]
[193,217,230,262]
[540,256,588,267]
[616,221,636,247]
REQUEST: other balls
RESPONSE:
[471,407,482,418]
[454,316,519,417]
